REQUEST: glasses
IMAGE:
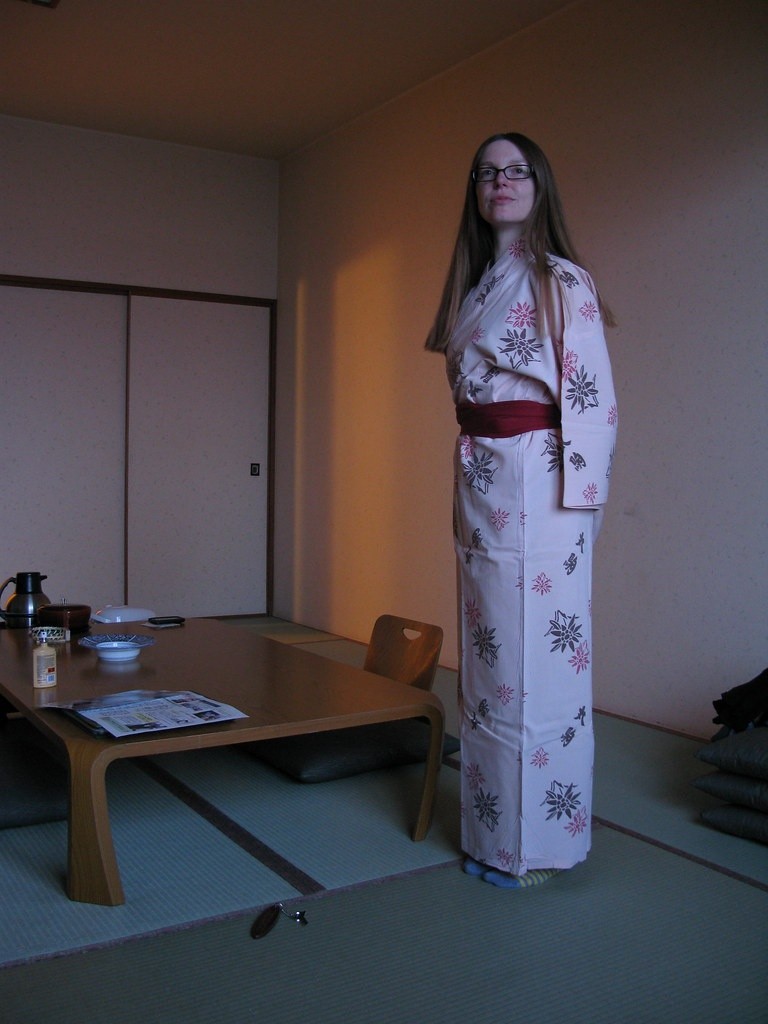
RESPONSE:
[472,164,533,182]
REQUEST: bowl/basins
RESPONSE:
[78,631,157,662]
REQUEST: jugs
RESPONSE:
[0,571,51,630]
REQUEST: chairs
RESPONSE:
[363,614,444,691]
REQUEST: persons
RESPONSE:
[423,131,618,889]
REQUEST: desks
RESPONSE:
[0,618,445,908]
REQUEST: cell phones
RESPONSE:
[149,615,186,625]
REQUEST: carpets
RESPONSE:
[0,699,464,970]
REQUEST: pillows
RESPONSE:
[241,718,461,784]
[689,725,768,843]
